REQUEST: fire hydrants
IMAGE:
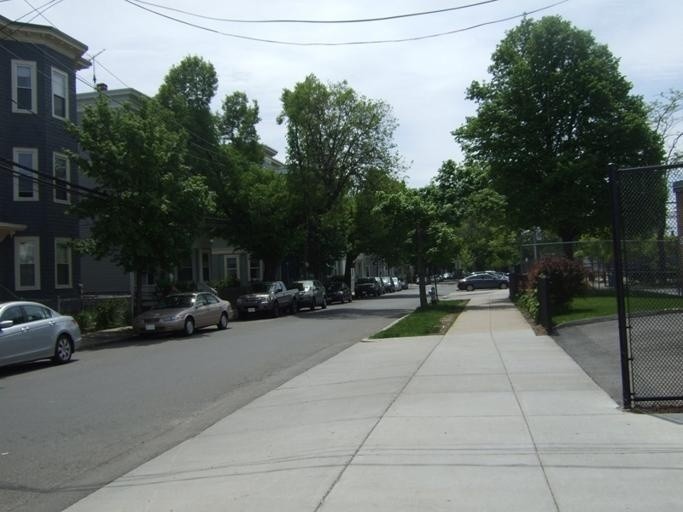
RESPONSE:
[428,287,436,304]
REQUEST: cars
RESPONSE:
[132,276,408,338]
[415,270,510,291]
[0,300,82,367]
